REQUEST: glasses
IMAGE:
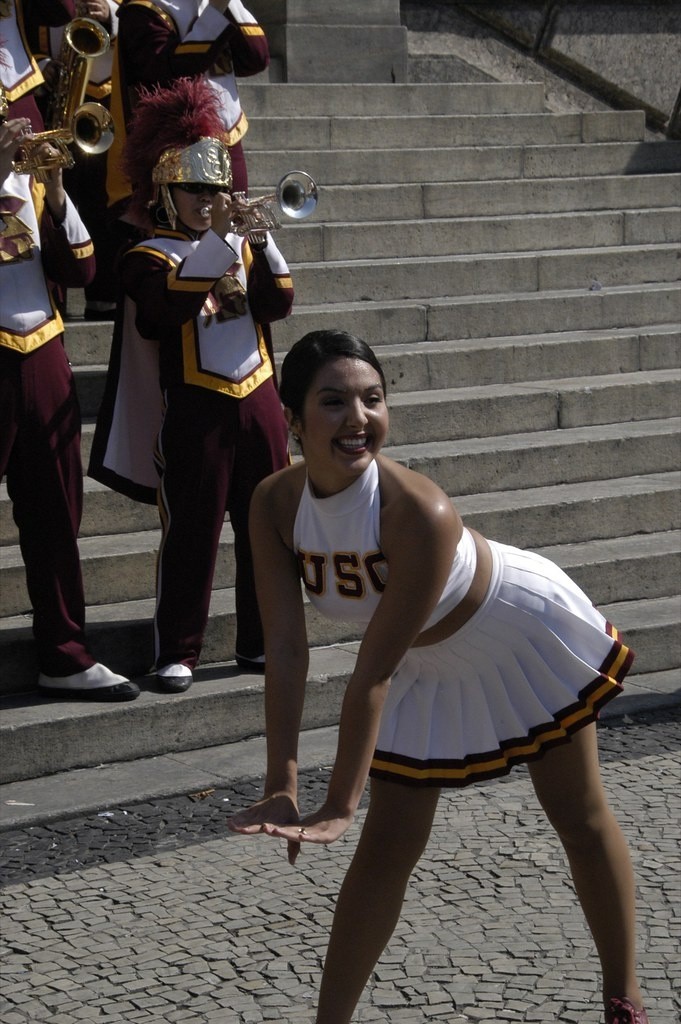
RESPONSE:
[172,182,228,197]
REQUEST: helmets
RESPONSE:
[125,73,233,232]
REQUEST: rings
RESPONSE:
[297,827,307,835]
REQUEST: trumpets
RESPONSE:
[10,101,116,184]
[201,170,319,245]
[46,18,111,145]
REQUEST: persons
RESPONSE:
[0,0,297,700]
[226,331,650,1024]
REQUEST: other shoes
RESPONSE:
[235,651,267,671]
[37,661,141,702]
[157,663,194,693]
